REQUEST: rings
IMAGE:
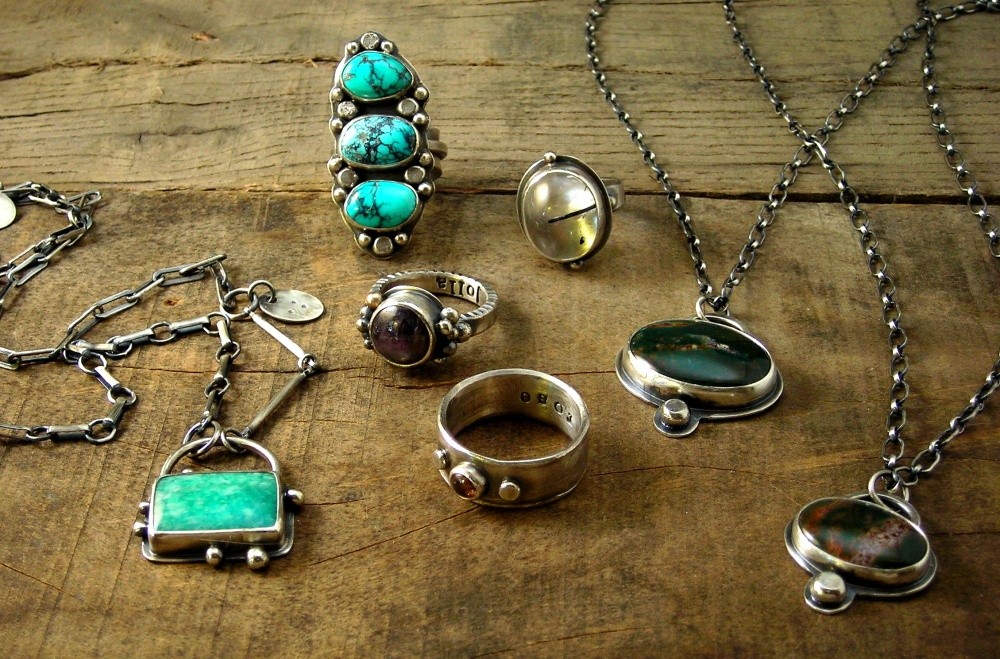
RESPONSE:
[357,269,497,371]
[434,368,591,508]
[328,31,448,263]
[516,151,625,269]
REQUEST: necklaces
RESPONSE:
[721,0,1000,614]
[1,179,327,567]
[582,0,1000,437]
